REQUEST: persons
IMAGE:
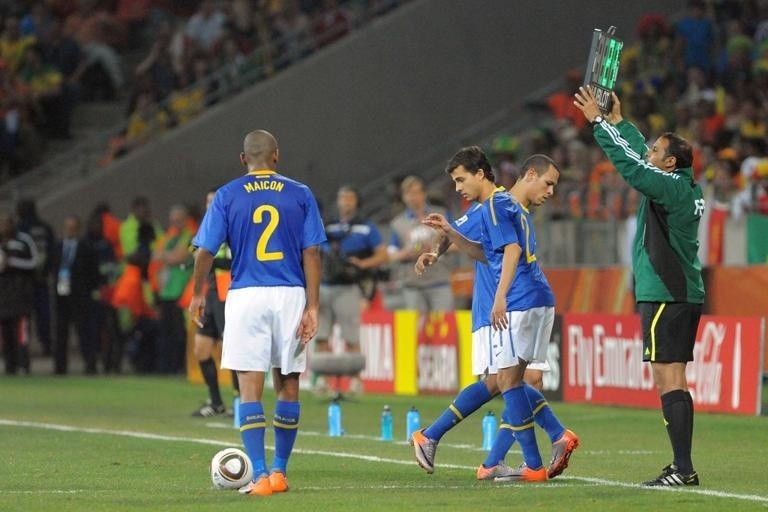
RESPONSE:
[425,146,578,484]
[1,193,190,377]
[572,83,704,486]
[388,171,455,312]
[409,154,560,483]
[189,185,239,418]
[311,185,388,406]
[1,0,189,192]
[441,4,768,212]
[189,129,328,496]
[190,2,374,123]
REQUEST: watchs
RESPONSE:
[590,114,604,126]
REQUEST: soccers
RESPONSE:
[209,448,252,491]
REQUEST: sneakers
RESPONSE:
[476,429,578,482]
[238,474,272,496]
[269,473,289,492]
[409,429,438,474]
[641,472,699,485]
[192,402,226,418]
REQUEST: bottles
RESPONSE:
[407,406,420,443]
[482,411,497,451]
[234,397,240,428]
[381,405,393,441]
[328,399,341,436]
